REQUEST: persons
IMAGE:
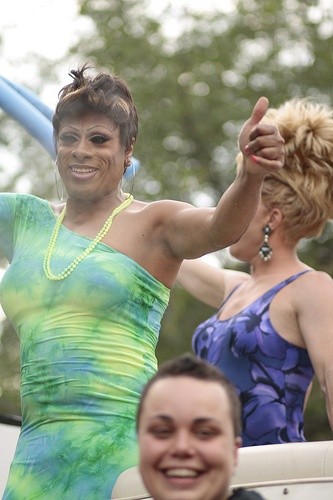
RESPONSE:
[134,351,266,500]
[0,60,286,500]
[175,92,333,448]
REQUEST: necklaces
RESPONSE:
[42,192,134,281]
